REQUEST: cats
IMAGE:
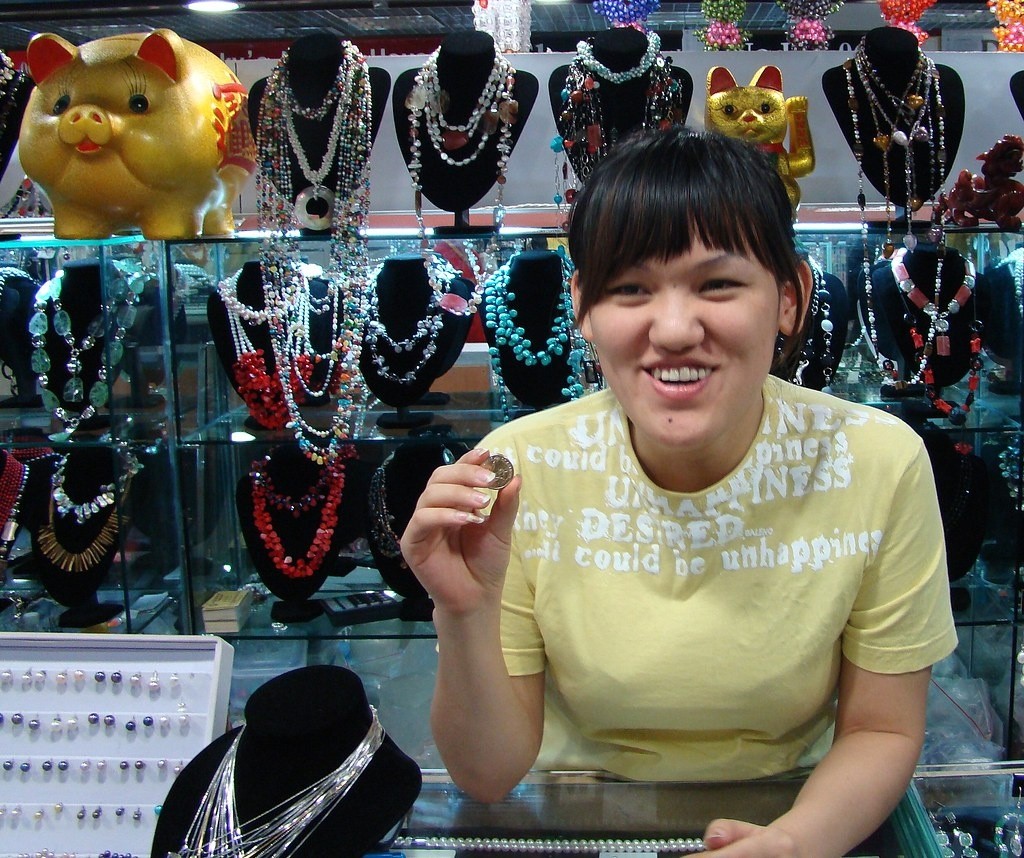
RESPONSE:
[704,65,816,217]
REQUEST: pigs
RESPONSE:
[15,28,258,239]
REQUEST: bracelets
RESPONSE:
[926,797,979,858]
[994,812,1024,858]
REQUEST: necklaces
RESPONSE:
[773,255,836,394]
[360,259,455,384]
[0,255,151,443]
[0,450,146,575]
[165,702,386,858]
[843,35,946,389]
[220,266,312,430]
[882,244,983,426]
[403,44,519,317]
[479,250,588,423]
[550,30,684,247]
[248,445,364,581]
[254,39,372,464]
[368,449,456,570]
[393,835,707,853]
[995,246,1024,319]
[999,435,1024,513]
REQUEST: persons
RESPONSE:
[397,121,959,858]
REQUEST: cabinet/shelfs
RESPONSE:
[0,234,232,635]
[156,223,1024,808]
